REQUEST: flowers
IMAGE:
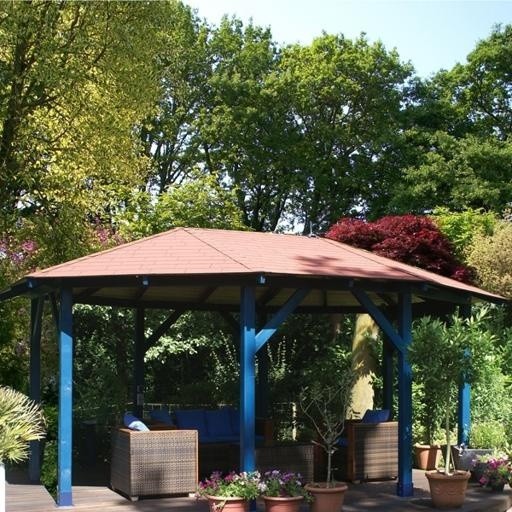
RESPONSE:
[258,469,316,506]
[470,455,512,488]
[195,470,261,510]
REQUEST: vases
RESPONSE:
[203,493,254,512]
[261,494,304,512]
[488,482,504,492]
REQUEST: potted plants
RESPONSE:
[451,417,505,479]
[298,380,348,512]
[403,306,499,509]
[412,383,443,471]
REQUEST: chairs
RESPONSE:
[111,420,199,501]
[345,420,399,484]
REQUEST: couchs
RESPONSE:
[141,418,284,480]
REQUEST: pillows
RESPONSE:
[174,408,210,444]
[360,409,390,422]
[123,413,150,431]
[207,406,238,442]
[150,407,174,425]
